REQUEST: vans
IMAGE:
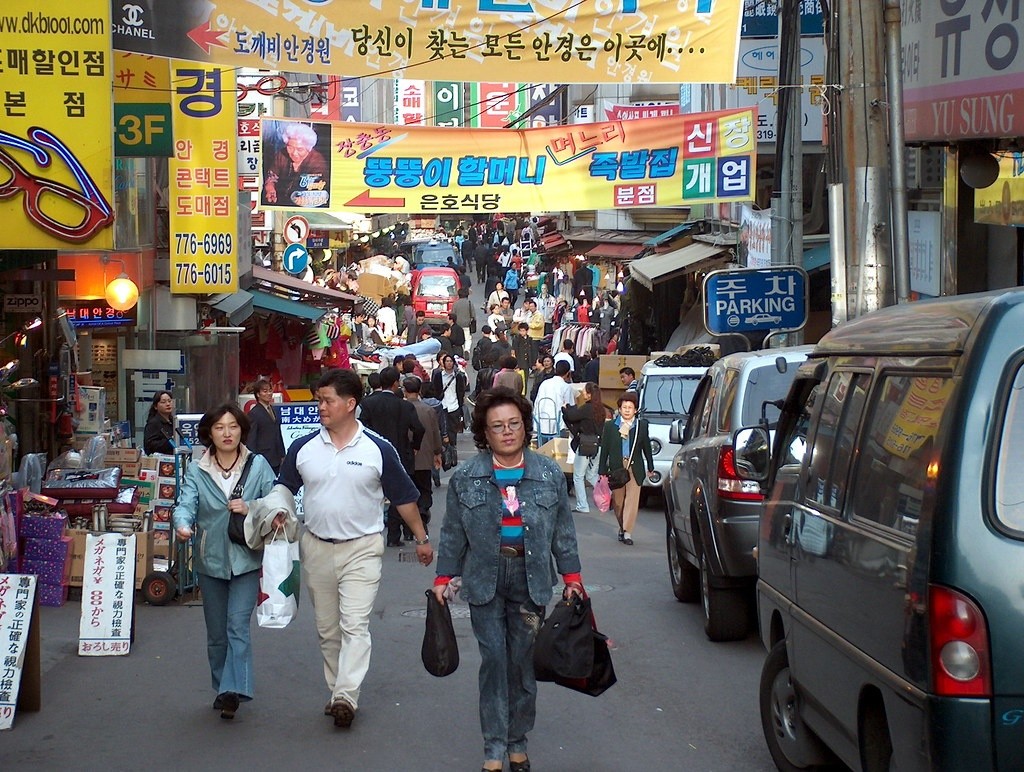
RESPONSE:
[631,357,712,508]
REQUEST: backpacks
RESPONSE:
[472,339,488,370]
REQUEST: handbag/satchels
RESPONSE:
[228,454,256,545]
[592,475,612,513]
[420,588,460,677]
[442,443,457,472]
[256,524,300,628]
[579,433,598,456]
[607,468,630,490]
[534,582,617,697]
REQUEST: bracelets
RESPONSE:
[413,534,429,546]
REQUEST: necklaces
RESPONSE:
[214,450,240,479]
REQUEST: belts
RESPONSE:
[500,546,525,557]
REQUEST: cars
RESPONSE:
[408,240,463,329]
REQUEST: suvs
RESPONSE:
[663,341,820,643]
[728,287,1024,772]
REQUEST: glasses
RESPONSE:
[487,419,524,433]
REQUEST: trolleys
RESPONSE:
[139,428,201,607]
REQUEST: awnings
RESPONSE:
[248,264,359,322]
[563,223,734,291]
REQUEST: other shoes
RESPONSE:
[508,752,530,772]
[330,697,354,728]
[622,537,633,545]
[618,530,624,541]
[213,695,222,709]
[404,535,414,542]
[220,692,239,719]
[572,509,580,513]
[324,698,331,714]
[482,761,503,772]
[467,396,476,406]
[387,540,406,547]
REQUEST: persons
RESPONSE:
[313,214,639,497]
[145,391,175,459]
[272,369,433,733]
[245,380,287,478]
[562,382,606,516]
[170,402,279,720]
[559,290,626,355]
[433,387,587,772]
[593,394,655,546]
[263,124,329,207]
